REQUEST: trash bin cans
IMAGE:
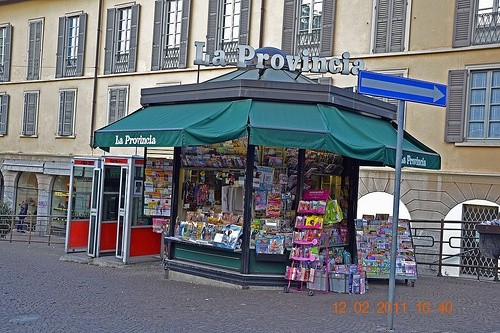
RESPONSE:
[475,219,500,258]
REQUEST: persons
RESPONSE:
[17,201,27,234]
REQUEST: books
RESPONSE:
[139,139,415,299]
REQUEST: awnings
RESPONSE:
[91,99,443,171]
